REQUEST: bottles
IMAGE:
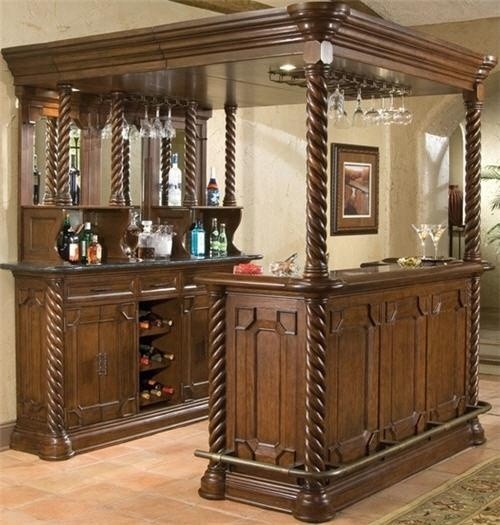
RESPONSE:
[206,166,220,206]
[139,307,176,399]
[168,151,183,206]
[68,154,81,205]
[53,213,103,265]
[188,214,228,258]
[118,208,175,261]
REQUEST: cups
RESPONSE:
[268,260,299,281]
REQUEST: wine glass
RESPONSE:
[412,222,449,260]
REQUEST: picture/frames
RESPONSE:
[329,142,381,237]
